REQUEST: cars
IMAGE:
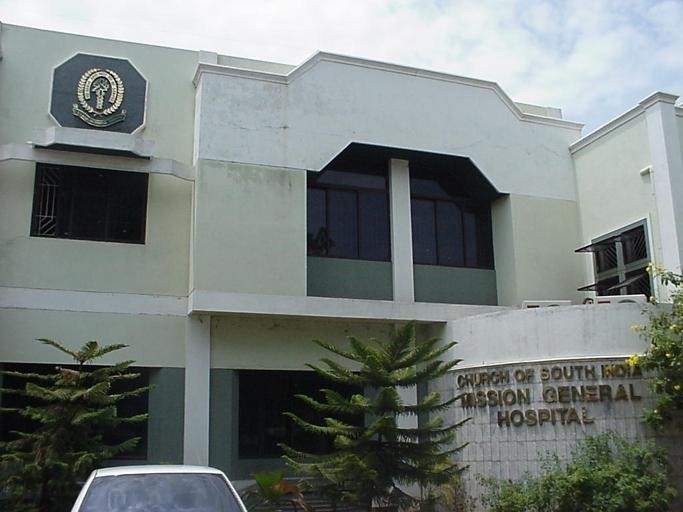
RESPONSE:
[68,463,249,512]
